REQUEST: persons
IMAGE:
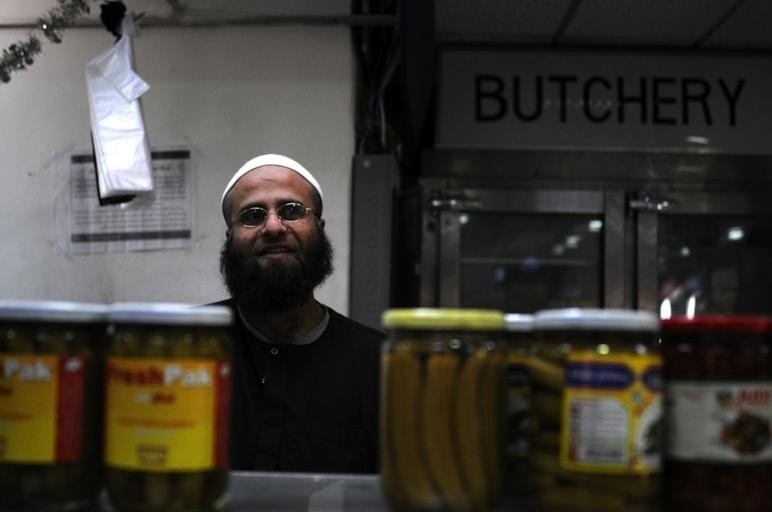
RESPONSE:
[90,154,389,512]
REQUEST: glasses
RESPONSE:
[229,203,318,229]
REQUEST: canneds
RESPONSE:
[378,308,507,510]
[2,302,105,511]
[495,314,536,511]
[530,307,662,510]
[105,302,232,511]
[661,317,770,511]
